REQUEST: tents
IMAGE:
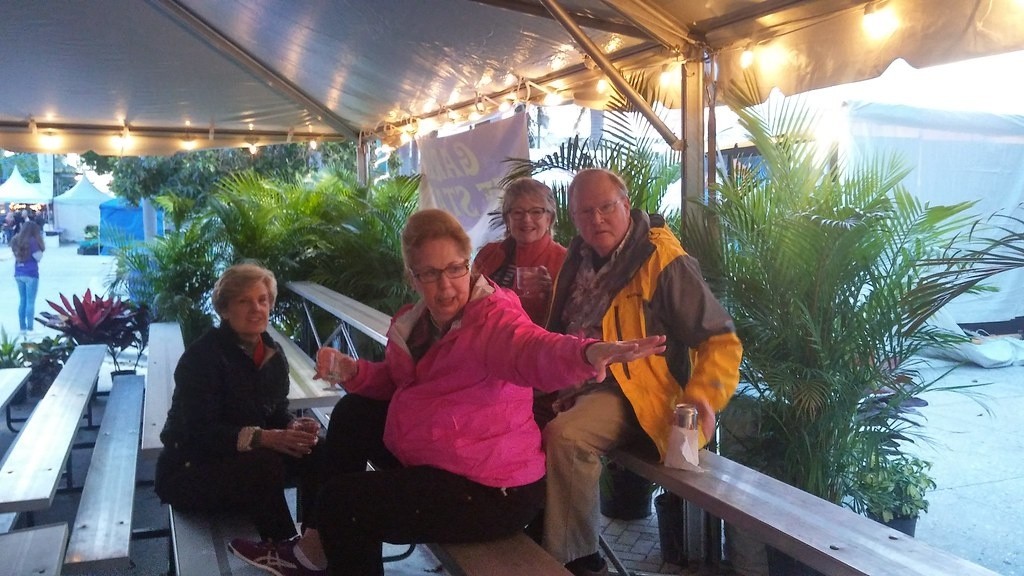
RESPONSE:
[98,196,166,257]
[0,164,50,224]
[53,171,114,228]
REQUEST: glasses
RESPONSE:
[509,207,551,220]
[409,259,471,284]
[571,199,624,217]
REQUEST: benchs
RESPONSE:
[0,247,1024,576]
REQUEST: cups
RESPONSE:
[516,266,539,295]
[293,420,321,446]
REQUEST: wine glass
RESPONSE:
[319,352,349,391]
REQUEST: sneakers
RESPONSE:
[227,536,327,576]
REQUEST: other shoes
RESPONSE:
[20,329,38,335]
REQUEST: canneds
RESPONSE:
[673,403,698,430]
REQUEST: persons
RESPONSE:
[153,263,327,542]
[228,170,743,576]
[11,221,45,336]
[0,210,44,245]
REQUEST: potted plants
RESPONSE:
[493,128,1009,576]
[0,218,231,403]
